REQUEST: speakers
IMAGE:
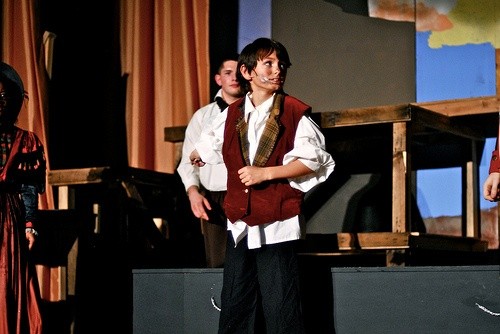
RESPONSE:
[131,269,226,333]
[328,263,500,334]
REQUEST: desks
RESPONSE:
[164,94,500,268]
[45,165,181,334]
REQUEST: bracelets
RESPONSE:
[25,228,38,235]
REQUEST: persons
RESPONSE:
[177,53,241,268]
[484,137,500,202]
[189,37,336,334]
[0,63,47,334]
